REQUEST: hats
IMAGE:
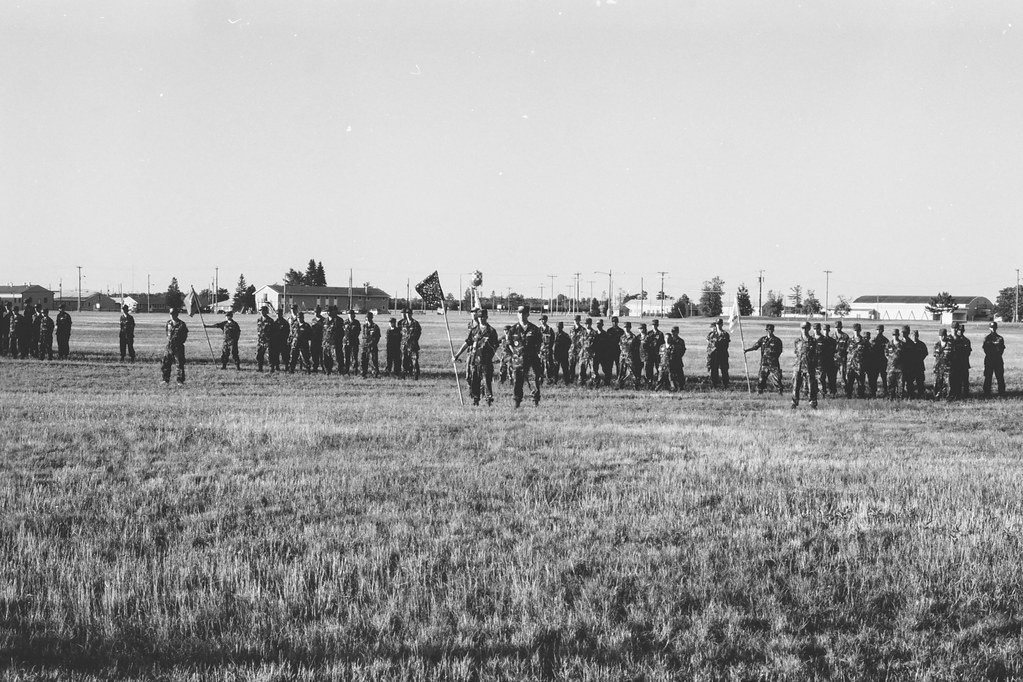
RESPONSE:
[938,329,947,336]
[406,309,413,314]
[276,309,283,315]
[469,306,680,344]
[225,312,233,317]
[400,308,406,313]
[958,325,965,330]
[122,305,128,309]
[259,306,269,314]
[710,322,716,328]
[170,307,178,316]
[313,303,359,321]
[365,312,373,317]
[951,321,959,328]
[389,318,396,322]
[716,319,723,324]
[297,312,304,318]
[4,297,67,317]
[290,304,298,309]
[988,322,997,328]
[765,325,775,330]
[799,317,920,345]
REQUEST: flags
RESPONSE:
[414,269,445,305]
[727,291,740,334]
[183,288,202,317]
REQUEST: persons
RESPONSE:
[159,307,188,387]
[507,306,543,410]
[496,324,515,386]
[386,307,422,380]
[705,319,730,389]
[982,322,1006,396]
[744,324,786,395]
[119,305,136,363]
[789,320,973,410]
[0,298,71,361]
[201,311,241,372]
[538,314,686,392]
[255,304,381,378]
[451,306,499,407]
